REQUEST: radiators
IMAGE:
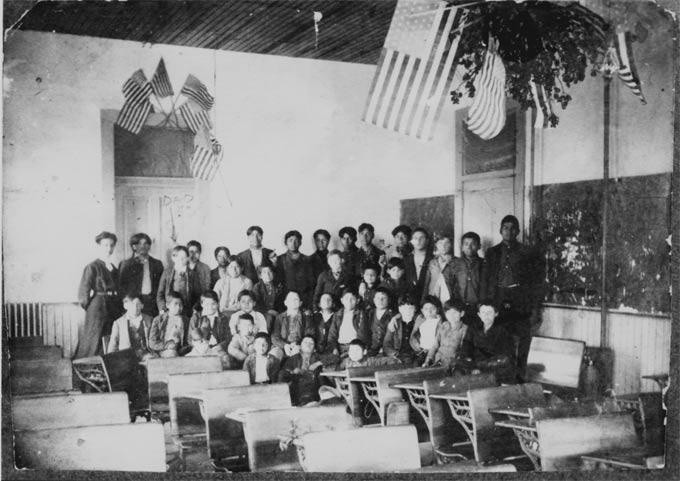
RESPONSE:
[5,301,87,358]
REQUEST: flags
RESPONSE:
[179,72,215,111]
[529,38,567,128]
[361,1,470,143]
[115,97,152,134]
[188,122,223,182]
[463,30,511,143]
[121,69,151,108]
[148,58,174,98]
[179,98,212,132]
[568,20,647,107]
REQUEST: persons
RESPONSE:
[73,230,117,359]
[106,223,513,386]
[487,215,547,386]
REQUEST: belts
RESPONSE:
[96,291,117,295]
